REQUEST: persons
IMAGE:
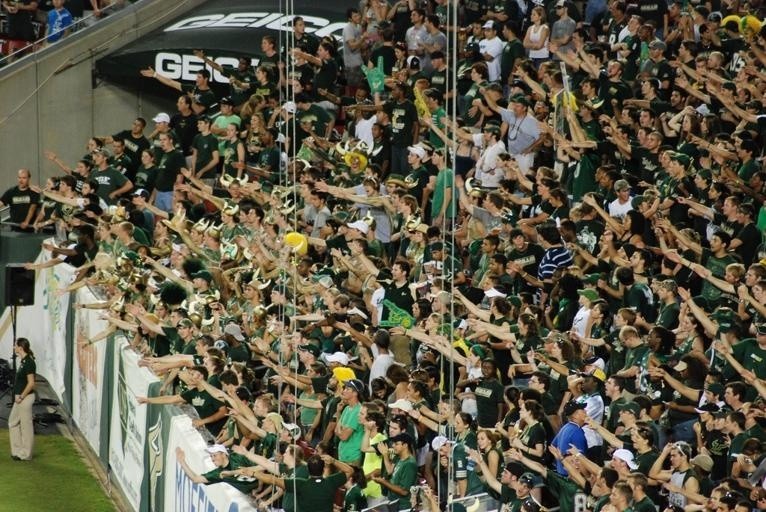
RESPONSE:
[1,0,766,512]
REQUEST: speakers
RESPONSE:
[6,267,36,306]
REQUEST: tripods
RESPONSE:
[0,307,23,407]
